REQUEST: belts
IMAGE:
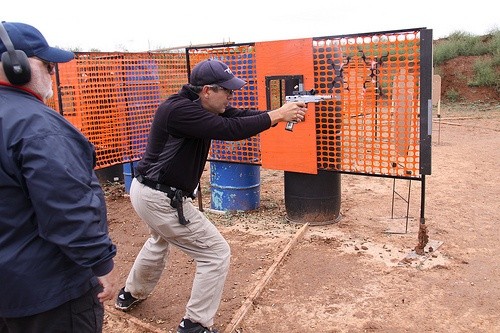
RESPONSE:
[136,174,190,197]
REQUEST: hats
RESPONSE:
[190,58,247,90]
[0,21,75,63]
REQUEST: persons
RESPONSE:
[115,58,308,333]
[0,22,118,333]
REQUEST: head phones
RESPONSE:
[0,23,31,85]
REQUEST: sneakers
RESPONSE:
[176,318,211,333]
[114,287,145,310]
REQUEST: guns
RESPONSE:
[285,89,333,132]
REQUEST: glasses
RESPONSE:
[213,88,233,94]
[32,57,56,74]
